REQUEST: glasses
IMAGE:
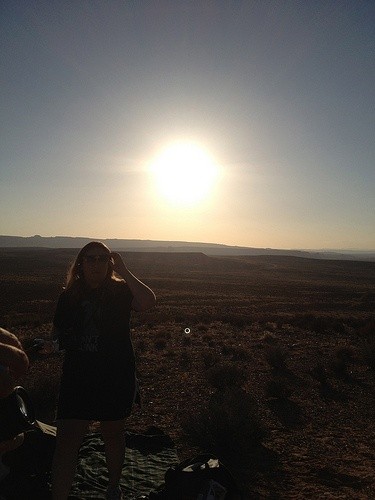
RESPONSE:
[82,255,109,262]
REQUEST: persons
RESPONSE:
[0,323,31,460]
[46,239,157,500]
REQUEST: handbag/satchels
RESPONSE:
[164,454,236,500]
